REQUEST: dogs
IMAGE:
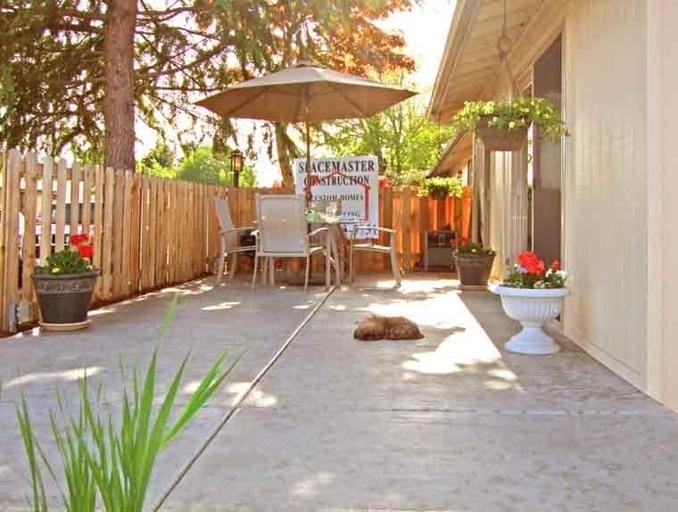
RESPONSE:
[353,316,425,341]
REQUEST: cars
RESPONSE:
[32,197,97,264]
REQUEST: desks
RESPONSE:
[254,215,359,290]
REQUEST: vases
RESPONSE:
[496,289,569,355]
[470,118,531,151]
[33,274,98,332]
[454,256,495,285]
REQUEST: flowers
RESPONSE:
[459,100,565,130]
[451,236,493,254]
[29,234,102,273]
[501,252,566,289]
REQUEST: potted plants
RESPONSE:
[412,178,461,197]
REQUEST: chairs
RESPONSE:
[250,192,331,293]
[311,199,342,247]
[349,201,411,286]
[213,194,273,287]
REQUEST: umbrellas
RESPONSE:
[192,62,420,207]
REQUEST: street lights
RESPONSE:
[230,149,246,187]
